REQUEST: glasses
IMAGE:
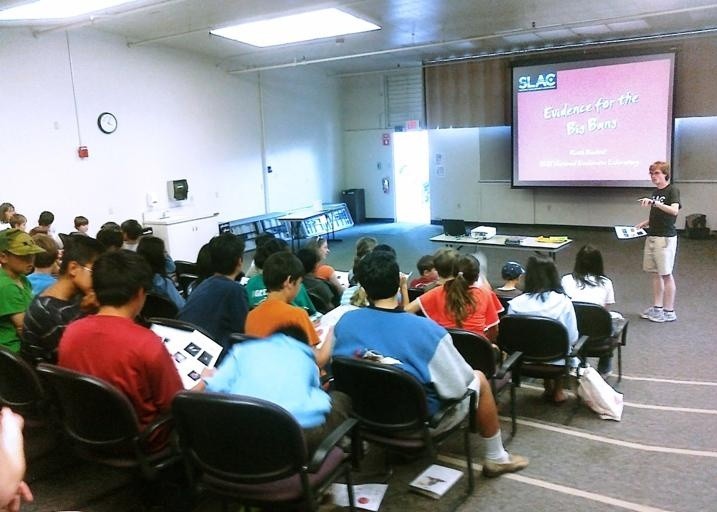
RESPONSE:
[316,235,323,242]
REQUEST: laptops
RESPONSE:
[441,219,466,237]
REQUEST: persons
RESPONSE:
[0,202,624,512]
[633,161,681,322]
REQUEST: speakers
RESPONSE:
[341,189,365,223]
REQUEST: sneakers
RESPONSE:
[483,450,529,478]
[640,306,663,319]
[554,389,576,404]
[599,371,613,381]
[569,363,590,377]
[337,439,369,456]
[649,310,676,322]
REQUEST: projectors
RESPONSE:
[470,225,497,241]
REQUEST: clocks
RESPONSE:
[97,112,118,134]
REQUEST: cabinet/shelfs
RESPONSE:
[142,207,220,264]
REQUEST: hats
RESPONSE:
[502,262,525,277]
[1,228,47,256]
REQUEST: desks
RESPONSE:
[277,202,354,255]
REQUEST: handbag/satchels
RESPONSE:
[577,367,624,422]
